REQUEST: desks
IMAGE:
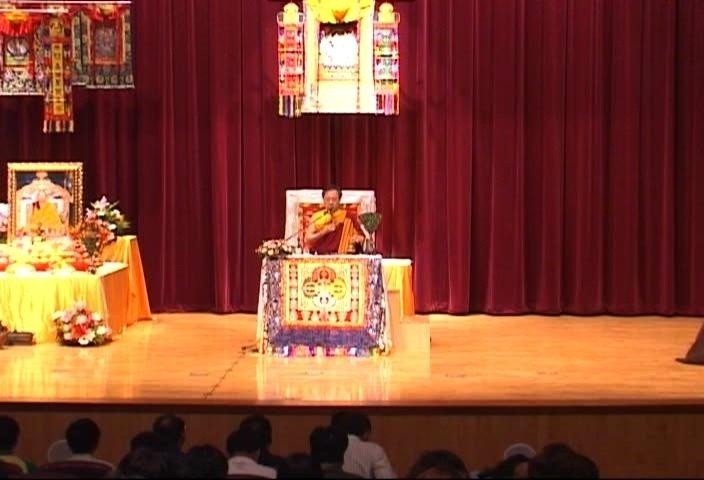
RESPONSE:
[0,261,129,345]
[256,253,393,358]
[101,235,152,327]
[380,259,414,321]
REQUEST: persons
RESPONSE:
[303,185,372,255]
[0,410,601,480]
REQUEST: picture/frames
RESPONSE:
[7,161,84,244]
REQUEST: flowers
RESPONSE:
[255,239,297,259]
[72,210,116,272]
[90,196,130,236]
[50,302,111,346]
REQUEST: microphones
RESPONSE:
[282,207,333,241]
